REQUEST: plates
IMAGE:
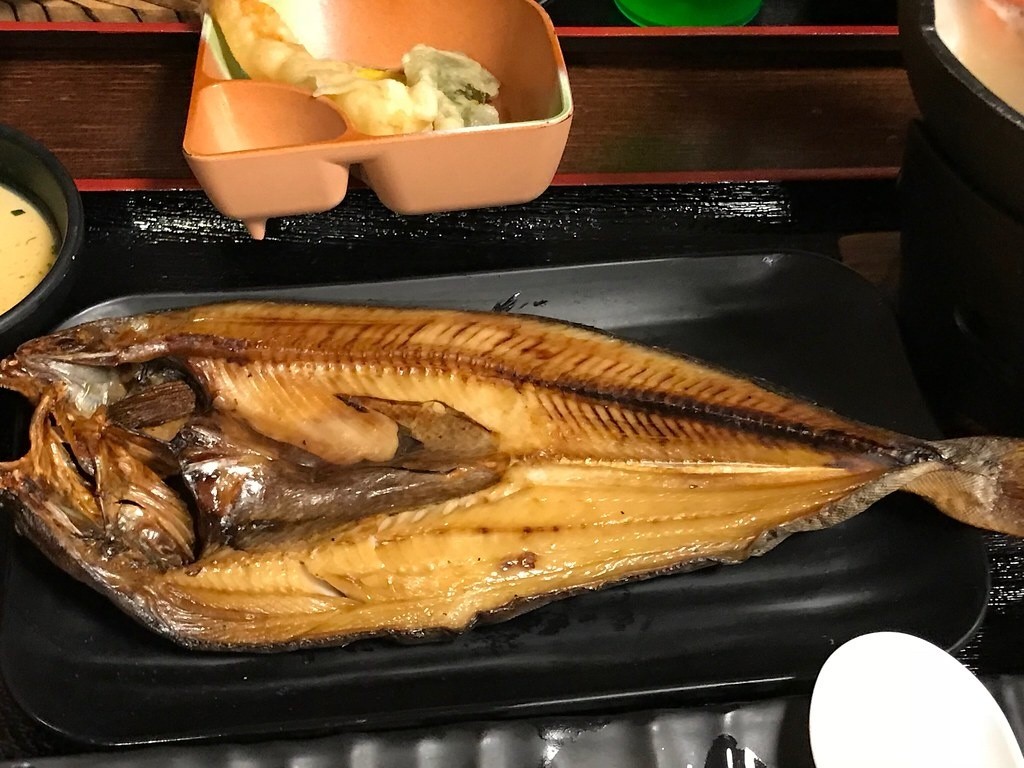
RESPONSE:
[0,253,988,752]
[808,632,1024,768]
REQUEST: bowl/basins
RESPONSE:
[0,122,83,335]
